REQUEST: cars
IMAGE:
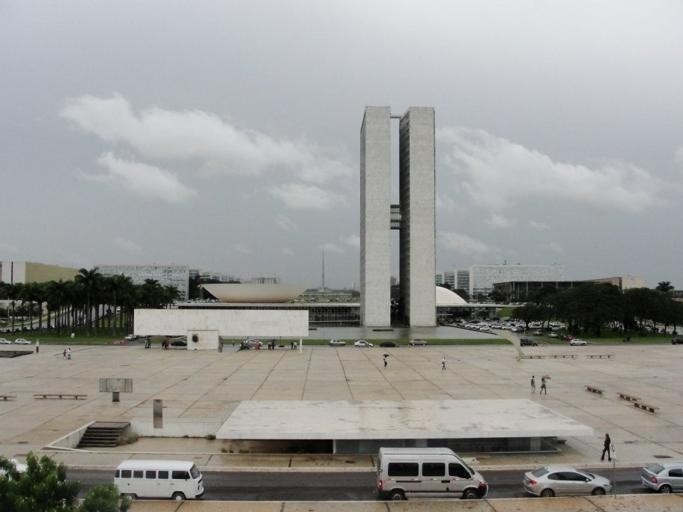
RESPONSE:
[0,459,29,481]
[171,341,187,346]
[409,338,428,347]
[523,464,613,497]
[354,340,374,347]
[640,463,683,493]
[0,338,11,344]
[455,320,587,347]
[379,341,398,347]
[329,339,346,345]
[240,339,263,348]
[14,338,31,345]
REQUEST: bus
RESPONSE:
[112,461,205,500]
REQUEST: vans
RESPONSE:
[377,447,489,501]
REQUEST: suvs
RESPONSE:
[125,334,136,341]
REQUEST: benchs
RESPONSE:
[631,400,660,413]
[552,354,577,359]
[585,354,613,359]
[31,392,89,400]
[584,385,605,394]
[616,391,643,402]
[523,354,545,359]
[0,394,17,400]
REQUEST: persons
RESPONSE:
[530,375,536,392]
[34,338,40,353]
[539,377,547,395]
[440,358,448,371]
[236,338,298,352]
[62,347,71,360]
[382,353,391,368]
[599,434,613,461]
[144,336,170,350]
